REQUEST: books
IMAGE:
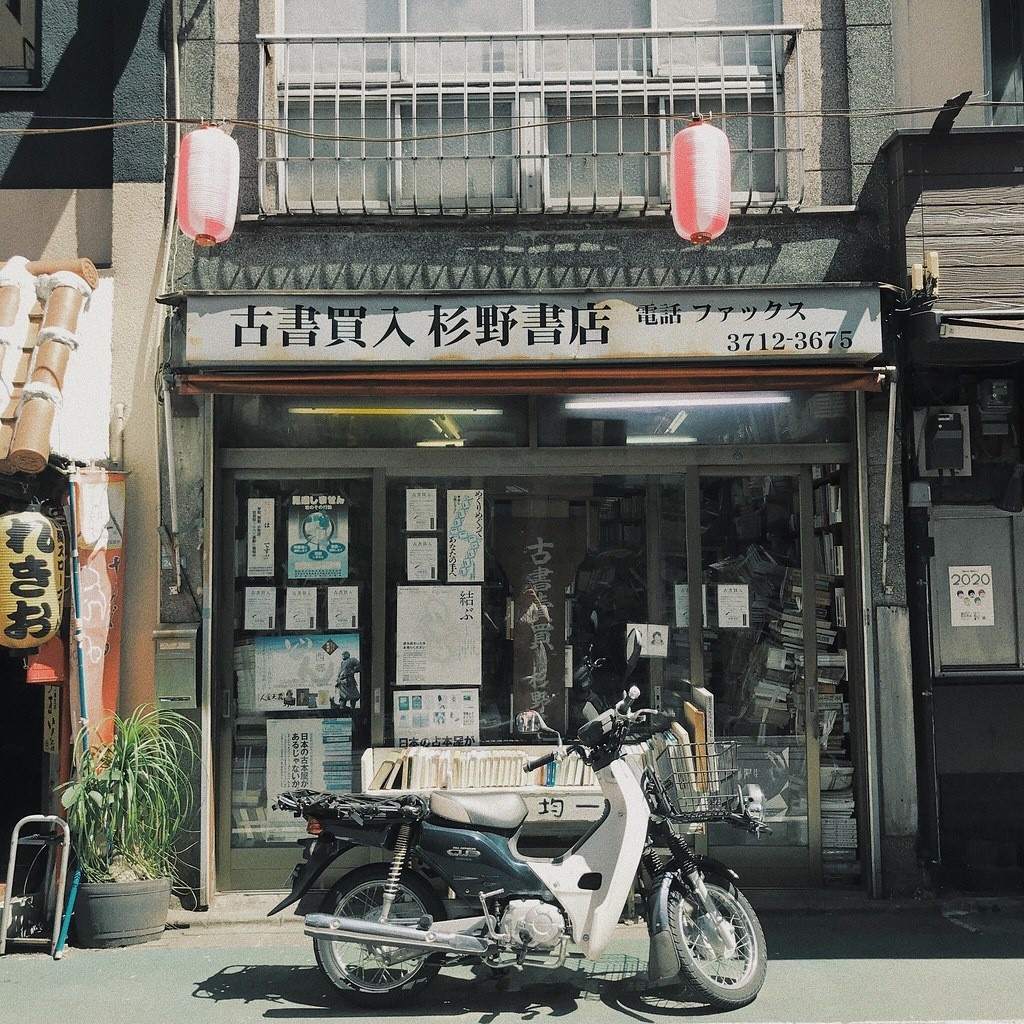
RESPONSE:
[479,464,858,864]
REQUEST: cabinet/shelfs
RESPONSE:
[492,494,602,737]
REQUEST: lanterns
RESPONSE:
[670,121,731,245]
[177,126,240,247]
[0,510,64,648]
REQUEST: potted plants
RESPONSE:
[49,700,208,951]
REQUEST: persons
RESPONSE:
[651,631,664,646]
[335,651,362,709]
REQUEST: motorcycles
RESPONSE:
[261,627,770,1012]
